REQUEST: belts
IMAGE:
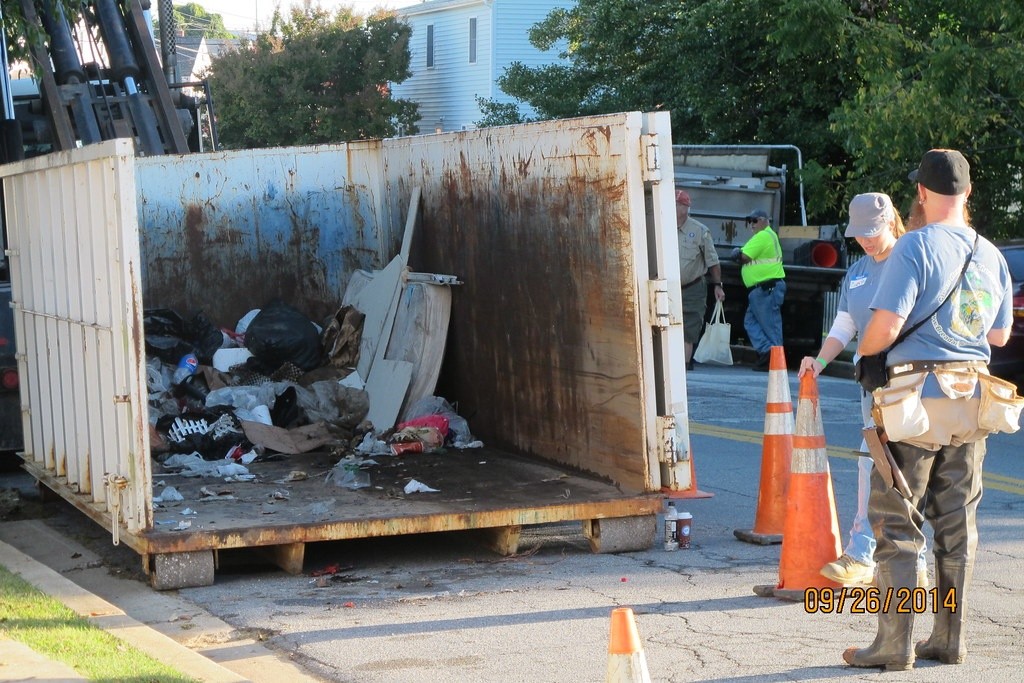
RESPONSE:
[752,278,782,289]
[681,275,702,289]
[889,358,988,379]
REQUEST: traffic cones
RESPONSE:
[732,345,794,546]
[750,367,856,604]
[601,607,656,683]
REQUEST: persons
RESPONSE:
[730,209,786,371]
[799,192,930,592]
[676,190,725,370]
[843,148,1013,671]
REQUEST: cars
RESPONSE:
[985,244,1024,398]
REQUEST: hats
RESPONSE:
[908,149,970,195]
[844,193,894,237]
[675,189,691,206]
[745,209,768,219]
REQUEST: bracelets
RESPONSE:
[816,358,828,368]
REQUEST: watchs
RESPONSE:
[713,282,723,288]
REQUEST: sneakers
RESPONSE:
[918,570,928,588]
[821,554,873,584]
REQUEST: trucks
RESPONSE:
[672,144,855,361]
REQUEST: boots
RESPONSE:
[914,436,986,664]
[843,442,939,670]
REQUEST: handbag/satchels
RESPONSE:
[853,351,888,397]
[692,298,733,365]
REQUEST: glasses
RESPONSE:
[748,218,762,224]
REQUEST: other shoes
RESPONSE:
[755,350,770,365]
[752,363,769,372]
